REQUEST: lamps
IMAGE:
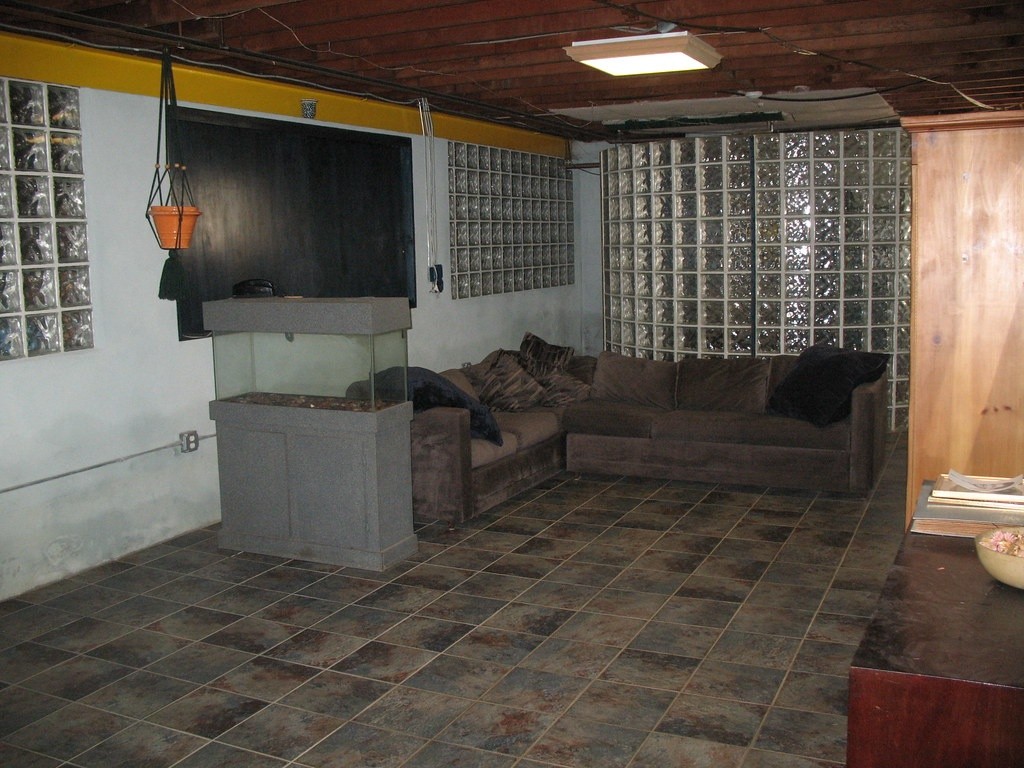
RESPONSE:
[561,32,725,76]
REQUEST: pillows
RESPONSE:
[479,347,547,414]
[590,350,677,410]
[537,361,592,407]
[520,332,575,377]
[767,345,893,430]
[675,358,771,416]
[368,365,504,449]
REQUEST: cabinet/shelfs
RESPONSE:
[845,480,1024,768]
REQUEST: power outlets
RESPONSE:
[180,431,201,453]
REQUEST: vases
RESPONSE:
[147,207,201,248]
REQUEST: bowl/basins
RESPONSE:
[975,526,1024,589]
[300,98,318,119]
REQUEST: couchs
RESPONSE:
[344,354,888,523]
